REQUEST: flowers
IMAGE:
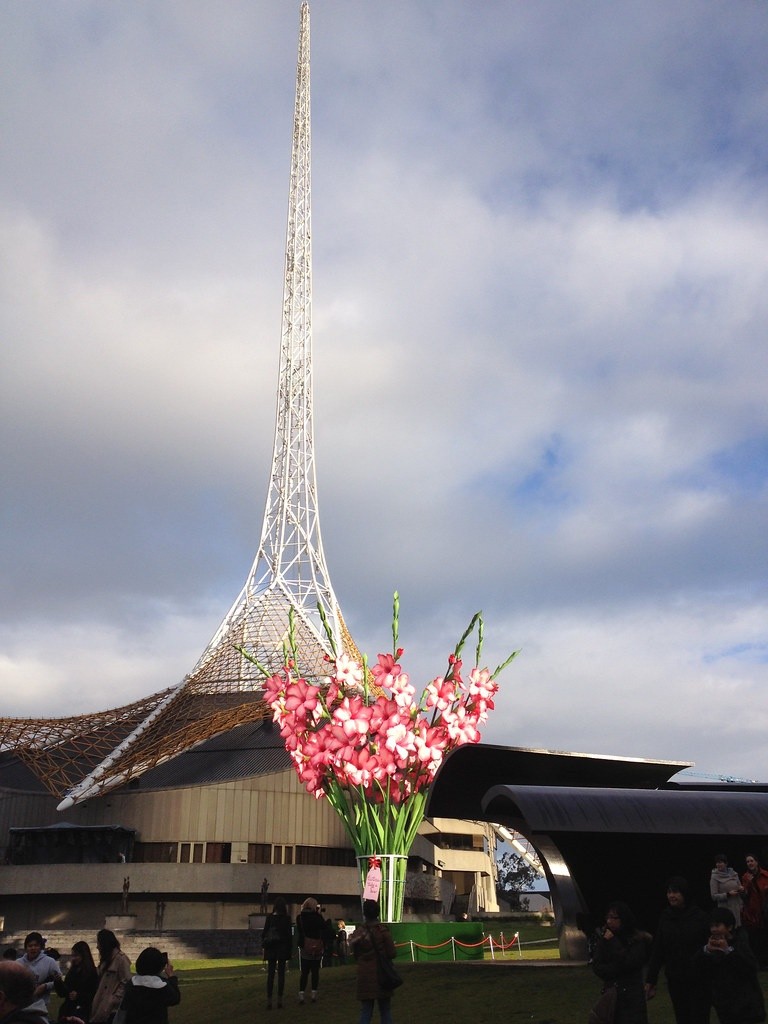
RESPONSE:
[232,591,524,921]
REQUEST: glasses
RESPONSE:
[605,915,619,921]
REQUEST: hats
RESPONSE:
[136,948,168,976]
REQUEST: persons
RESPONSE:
[644,877,765,1024]
[593,901,648,1024]
[261,897,347,1011]
[348,900,398,1024]
[710,852,768,972]
[0,929,181,1024]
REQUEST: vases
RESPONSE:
[355,854,409,922]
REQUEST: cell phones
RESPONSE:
[737,889,742,892]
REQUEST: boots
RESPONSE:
[298,991,304,1004]
[311,991,317,1001]
[267,996,272,1010]
[277,996,282,1008]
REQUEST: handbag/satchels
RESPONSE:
[303,937,324,956]
[367,926,404,992]
[261,916,280,949]
[739,906,760,928]
[588,987,616,1024]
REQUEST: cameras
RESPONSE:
[710,935,721,940]
[317,907,325,912]
[162,952,168,963]
